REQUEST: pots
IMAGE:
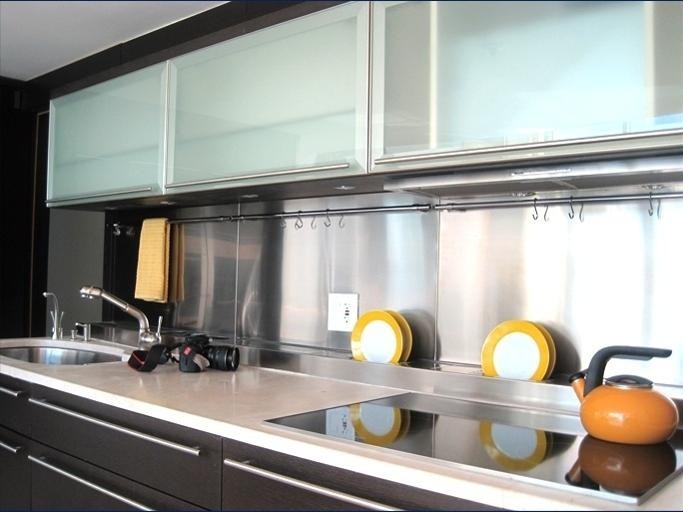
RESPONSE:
[567,345,679,445]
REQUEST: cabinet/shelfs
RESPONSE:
[0,371,516,512]
[369,0,683,172]
[163,0,369,207]
[45,61,166,214]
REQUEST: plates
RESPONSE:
[350,309,412,365]
[479,419,553,471]
[480,319,557,382]
[350,402,410,447]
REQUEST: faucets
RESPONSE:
[78,284,150,343]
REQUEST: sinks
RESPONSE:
[2,337,127,365]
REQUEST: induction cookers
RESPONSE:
[263,391,682,506]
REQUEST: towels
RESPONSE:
[134,217,185,306]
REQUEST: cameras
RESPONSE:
[179,335,240,372]
